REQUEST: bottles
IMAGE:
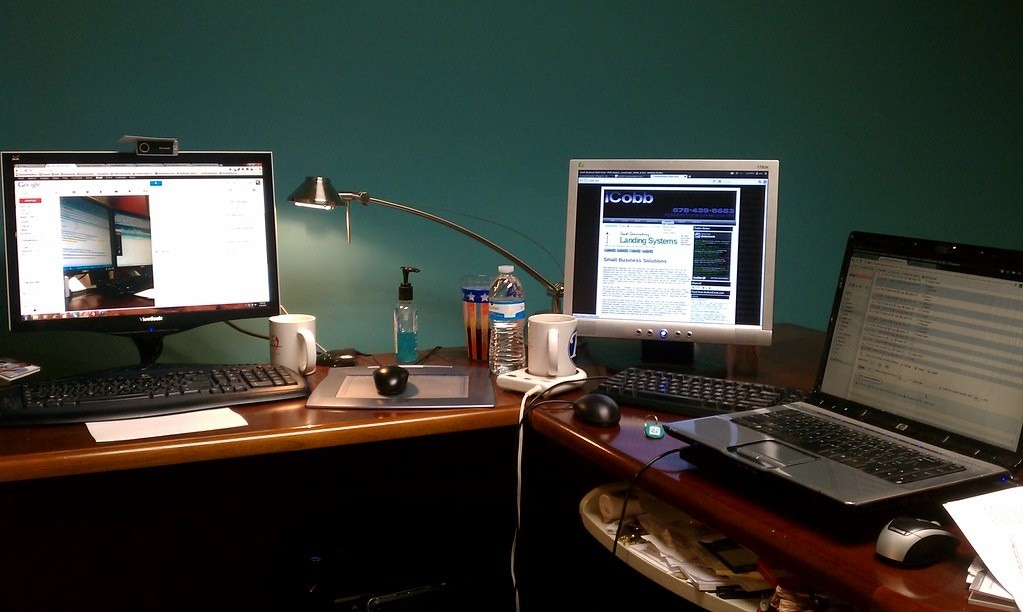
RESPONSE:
[485,264,528,375]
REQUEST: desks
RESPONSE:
[1,322,1018,612]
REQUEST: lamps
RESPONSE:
[288,176,567,318]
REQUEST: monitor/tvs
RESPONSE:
[1,150,280,366]
[563,157,780,369]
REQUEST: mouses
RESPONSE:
[572,394,620,428]
[373,364,409,397]
[875,517,960,568]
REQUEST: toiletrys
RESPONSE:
[393,266,421,363]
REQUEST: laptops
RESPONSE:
[662,232,1022,523]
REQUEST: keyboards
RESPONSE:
[0,363,312,427]
[591,366,812,418]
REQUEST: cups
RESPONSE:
[526,310,578,377]
[461,286,503,362]
[269,313,318,377]
